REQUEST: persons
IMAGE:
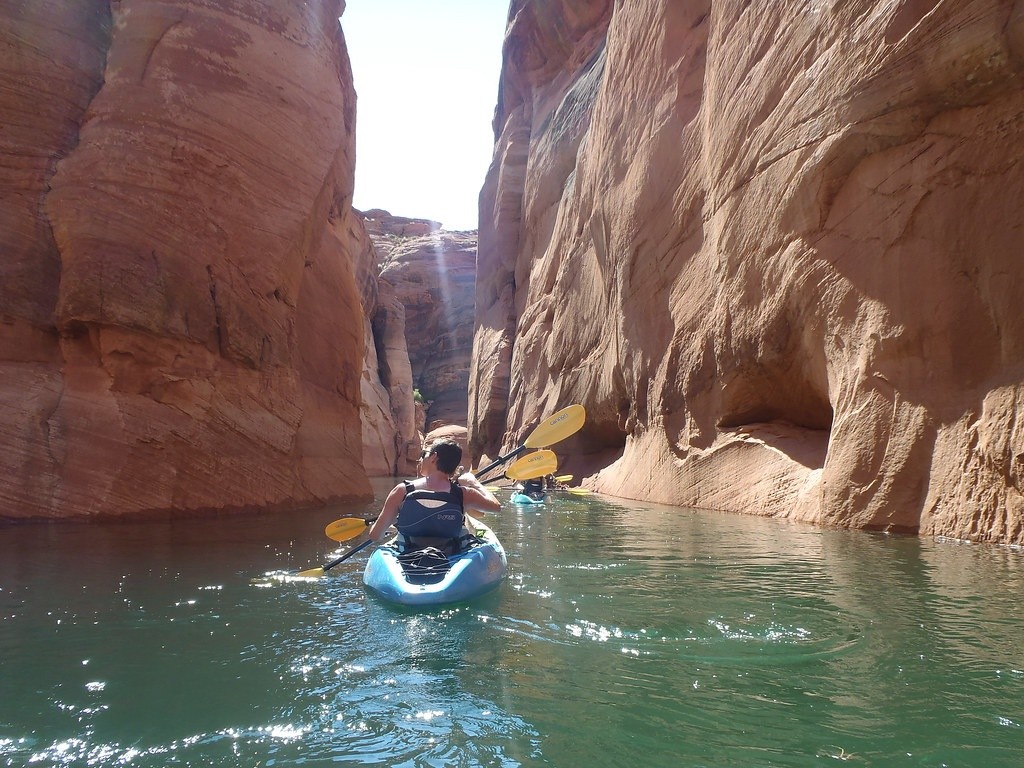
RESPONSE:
[512,475,548,497]
[368,436,502,561]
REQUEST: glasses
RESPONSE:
[421,450,436,459]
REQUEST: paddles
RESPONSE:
[324,448,559,543]
[555,474,573,482]
[484,486,590,496]
[295,403,588,577]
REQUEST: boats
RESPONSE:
[510,491,544,504]
[362,510,508,606]
